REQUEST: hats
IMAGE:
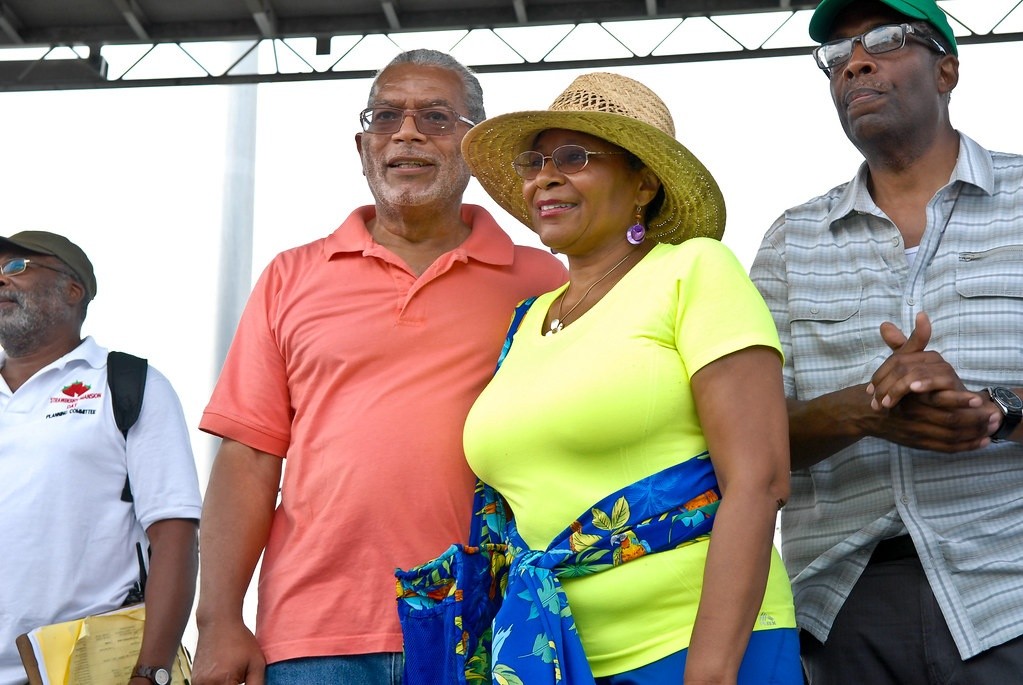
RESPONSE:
[809,0,959,58]
[0,230,97,300]
[460,72,727,245]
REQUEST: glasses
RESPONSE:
[0,258,78,281]
[812,23,947,81]
[360,107,477,136]
[513,145,645,180]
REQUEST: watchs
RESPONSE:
[980,385,1023,443]
[130,665,172,685]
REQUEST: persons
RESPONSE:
[193,49,806,685]
[0,230,200,685]
[746,0,1023,685]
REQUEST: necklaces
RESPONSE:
[544,255,631,337]
